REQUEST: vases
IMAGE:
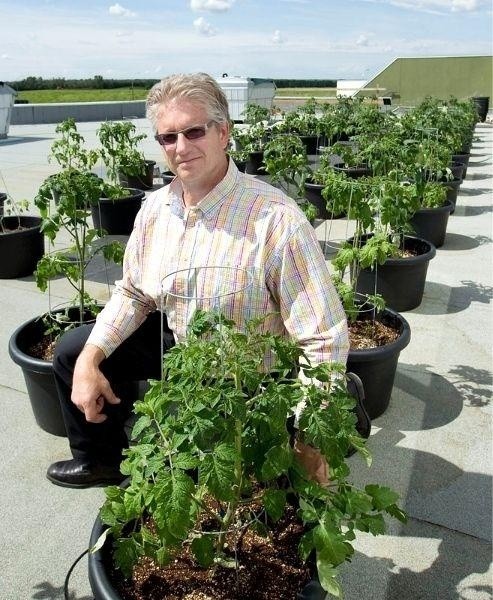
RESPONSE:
[471,97,490,122]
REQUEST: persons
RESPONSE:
[47,70,351,508]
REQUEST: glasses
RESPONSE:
[154,122,217,146]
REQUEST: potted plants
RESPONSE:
[87,308,409,599]
[0,93,483,435]
